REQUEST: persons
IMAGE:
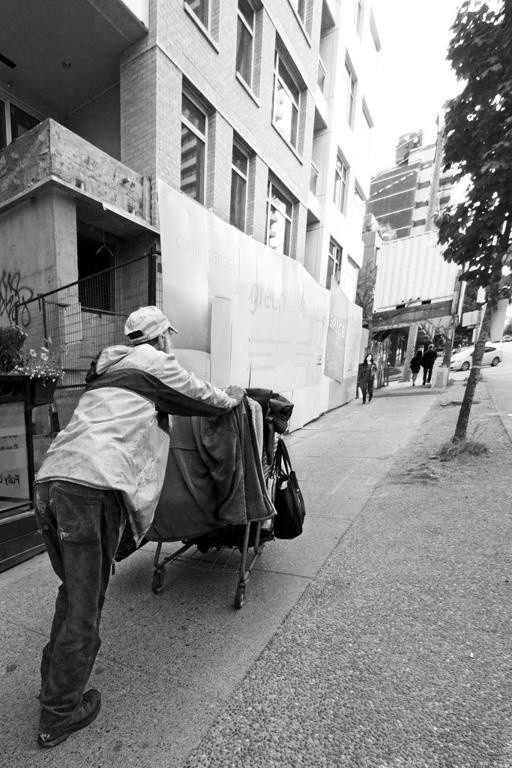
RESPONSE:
[33,305,249,749]
[357,353,377,404]
[409,344,438,386]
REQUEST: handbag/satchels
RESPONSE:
[261,471,306,539]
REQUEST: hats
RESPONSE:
[124,305,178,344]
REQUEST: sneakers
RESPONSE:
[37,689,101,748]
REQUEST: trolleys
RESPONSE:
[150,389,283,609]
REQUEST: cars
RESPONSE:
[441,346,503,371]
